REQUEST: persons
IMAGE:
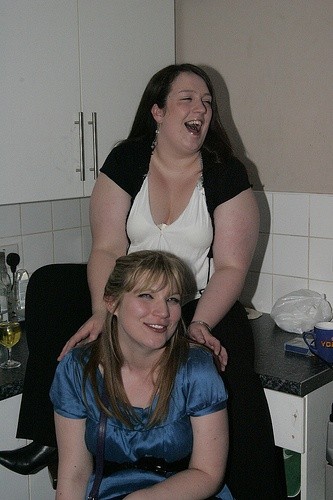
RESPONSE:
[50,251,234,500]
[57,63,288,500]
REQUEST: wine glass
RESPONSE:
[0,312,22,369]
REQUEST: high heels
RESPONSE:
[0,441,58,479]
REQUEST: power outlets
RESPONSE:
[0,243,20,272]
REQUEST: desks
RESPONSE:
[0,313,333,500]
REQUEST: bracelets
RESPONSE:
[190,320,212,332]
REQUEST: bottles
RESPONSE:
[0,251,13,327]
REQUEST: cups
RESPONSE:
[303,322,333,364]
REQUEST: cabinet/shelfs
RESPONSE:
[0,0,175,205]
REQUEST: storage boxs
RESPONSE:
[284,338,314,357]
